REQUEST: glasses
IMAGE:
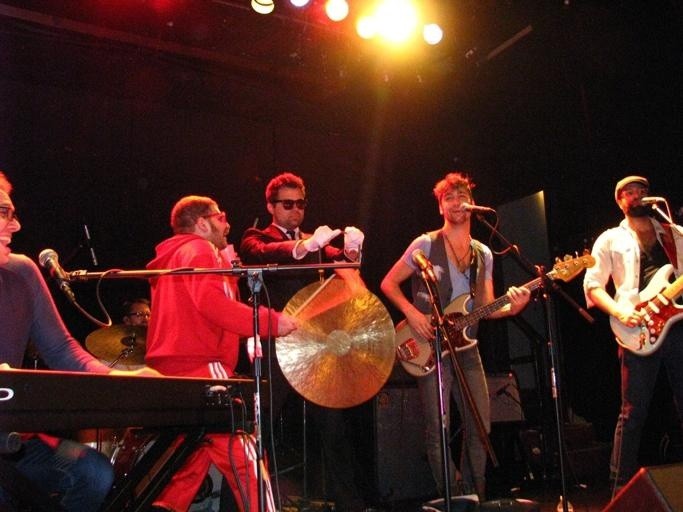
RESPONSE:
[0,206,16,220]
[273,198,307,210]
[202,210,226,220]
[128,311,150,317]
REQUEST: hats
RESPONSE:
[614,175,650,201]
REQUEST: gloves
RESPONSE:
[343,226,365,253]
[303,225,341,252]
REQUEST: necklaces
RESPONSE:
[444,231,473,274]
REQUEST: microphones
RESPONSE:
[411,248,437,283]
[460,202,491,213]
[39,248,77,302]
[490,383,509,398]
[640,196,663,205]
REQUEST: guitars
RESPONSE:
[609,263,683,355]
[395,249,594,376]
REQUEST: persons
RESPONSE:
[582,175,683,499]
[380,171,532,506]
[0,170,161,512]
[134,194,297,512]
[122,297,151,330]
[239,172,366,511]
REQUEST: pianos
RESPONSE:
[0,368,270,434]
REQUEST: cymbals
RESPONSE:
[85,325,148,362]
[275,280,394,409]
